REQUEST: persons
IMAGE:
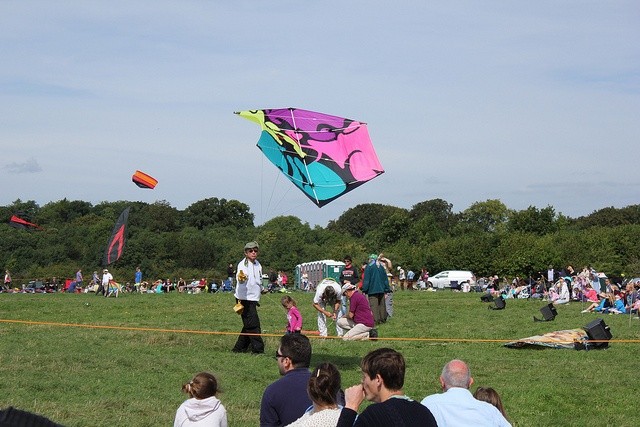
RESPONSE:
[393,265,436,292]
[361,253,391,324]
[336,347,439,427]
[357,261,368,290]
[173,372,228,427]
[281,295,303,335]
[283,362,359,427]
[340,255,360,315]
[267,269,316,293]
[0,263,236,298]
[420,359,512,427]
[451,262,640,317]
[473,386,506,417]
[312,277,342,337]
[337,283,378,342]
[259,332,346,427]
[231,241,267,354]
[377,253,393,318]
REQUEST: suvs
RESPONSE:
[417,271,476,291]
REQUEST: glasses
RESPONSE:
[344,260,349,262]
[274,351,292,359]
[248,250,257,253]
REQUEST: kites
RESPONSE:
[509,327,592,354]
[101,169,158,265]
[9,214,46,233]
[232,106,387,209]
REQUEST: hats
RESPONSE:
[341,283,356,295]
[344,255,352,261]
[244,242,259,250]
